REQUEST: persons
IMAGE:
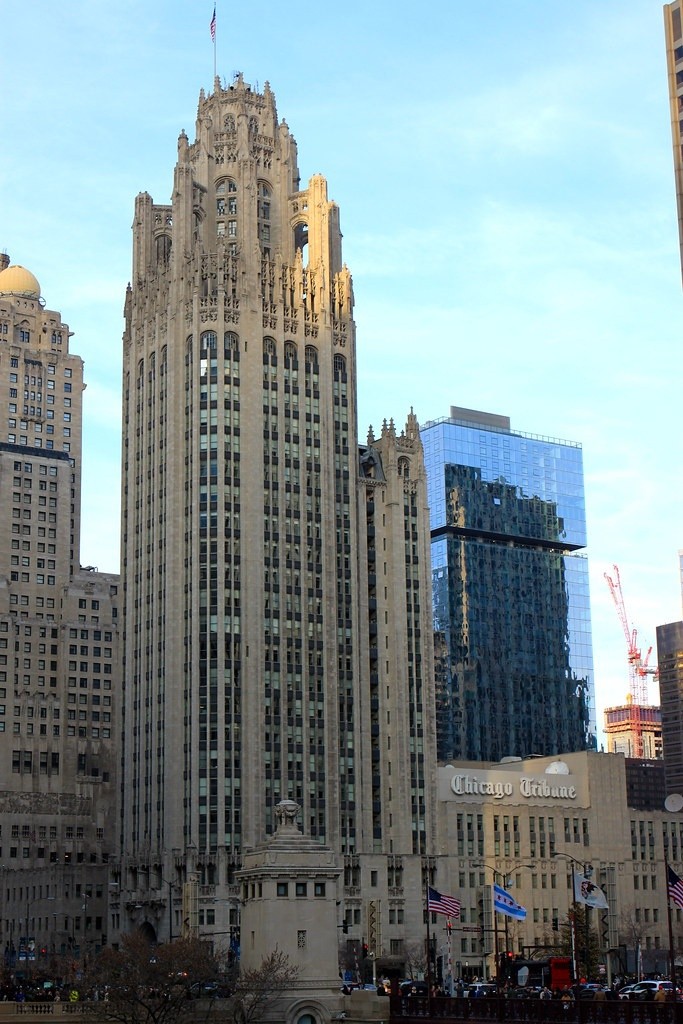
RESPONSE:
[0,975,160,1002]
[342,973,683,1019]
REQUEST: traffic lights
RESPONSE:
[363,944,368,957]
[342,920,346,933]
[508,951,512,957]
[41,948,46,953]
[552,918,558,931]
[446,920,452,936]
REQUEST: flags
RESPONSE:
[210,5,216,43]
[666,864,683,910]
[428,885,462,919]
[493,883,527,920]
[573,869,609,909]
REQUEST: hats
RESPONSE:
[659,985,662,990]
[412,987,416,989]
[382,977,389,982]
[598,985,601,989]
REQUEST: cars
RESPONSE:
[400,980,451,998]
[343,981,378,991]
[570,980,682,1000]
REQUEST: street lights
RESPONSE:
[549,851,590,981]
[23,896,56,979]
[137,869,204,944]
[53,911,75,985]
[471,862,537,956]
[83,881,119,975]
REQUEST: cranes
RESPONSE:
[604,563,659,761]
